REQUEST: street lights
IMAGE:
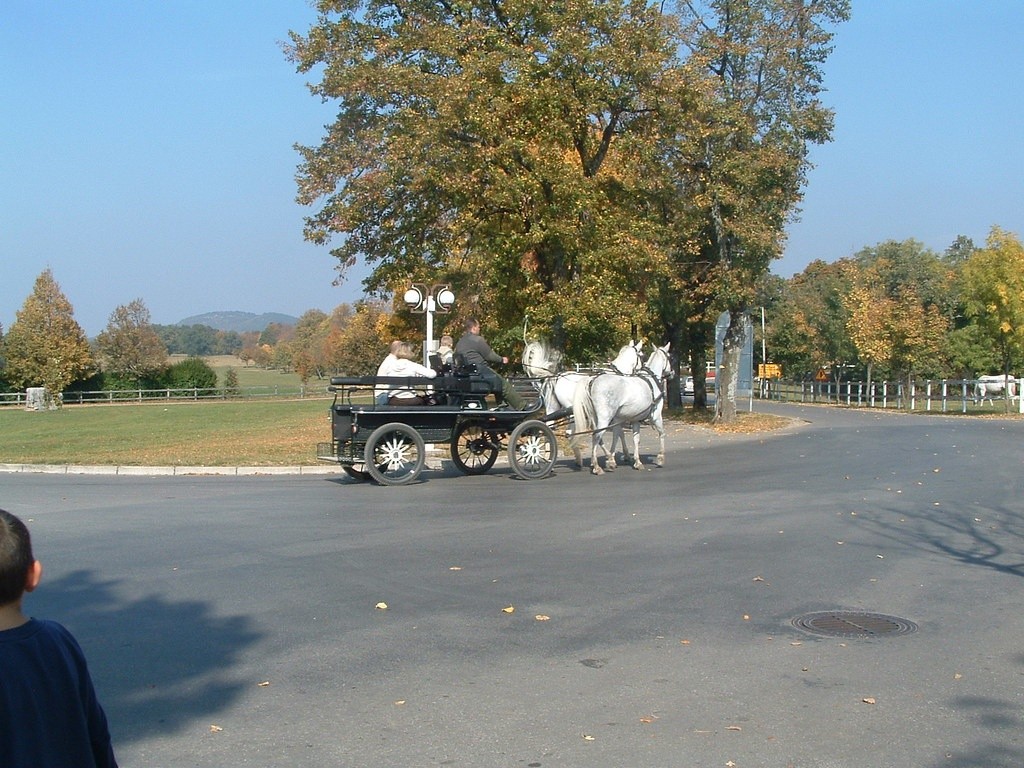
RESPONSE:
[403,282,455,396]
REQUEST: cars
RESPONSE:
[683,376,695,397]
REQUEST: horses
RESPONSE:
[545,339,675,475]
[970,374,1016,407]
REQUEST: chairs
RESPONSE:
[428,352,499,410]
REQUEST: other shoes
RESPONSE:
[523,399,540,411]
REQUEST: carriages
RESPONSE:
[315,338,675,487]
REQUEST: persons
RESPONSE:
[455,318,539,411]
[374,341,437,406]
[435,336,455,377]
[0,509,119,768]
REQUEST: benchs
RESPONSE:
[326,376,461,411]
